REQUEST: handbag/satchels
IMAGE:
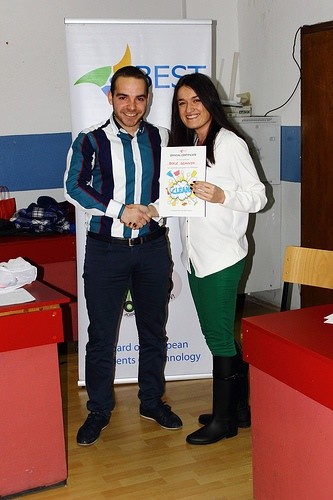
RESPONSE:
[0,185,17,222]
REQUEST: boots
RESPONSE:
[186,356,239,444]
[199,361,250,428]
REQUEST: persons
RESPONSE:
[120,73,268,445]
[63,66,183,446]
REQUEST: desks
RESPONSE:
[0,280,72,500]
[239,302,333,500]
[0,234,78,352]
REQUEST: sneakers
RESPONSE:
[77,412,109,445]
[140,401,183,429]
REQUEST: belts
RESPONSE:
[86,232,164,246]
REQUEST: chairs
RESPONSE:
[280,245,333,311]
